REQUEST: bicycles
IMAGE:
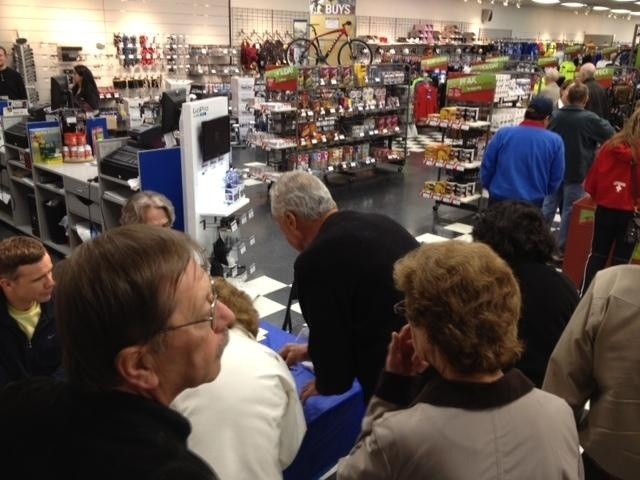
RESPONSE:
[284,19,375,72]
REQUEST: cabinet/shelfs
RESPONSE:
[1,41,255,300]
[255,41,541,223]
[538,39,638,133]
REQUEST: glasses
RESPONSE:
[391,299,414,316]
[146,270,218,337]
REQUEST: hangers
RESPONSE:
[234,29,295,44]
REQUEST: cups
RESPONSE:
[423,102,479,198]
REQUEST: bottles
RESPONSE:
[60,142,95,162]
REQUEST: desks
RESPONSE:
[248,317,368,480]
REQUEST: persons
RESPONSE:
[541,264,640,480]
[336,241,587,480]
[119,191,175,229]
[0,46,27,100]
[481,99,566,208]
[0,236,62,389]
[168,277,307,480]
[541,62,640,297]
[1,224,235,480]
[72,66,100,117]
[269,171,438,410]
[471,202,580,389]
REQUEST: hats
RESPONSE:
[529,91,556,119]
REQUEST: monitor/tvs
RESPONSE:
[162,88,186,134]
[51,76,73,110]
[202,114,230,162]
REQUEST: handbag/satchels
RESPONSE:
[626,218,640,244]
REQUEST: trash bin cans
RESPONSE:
[41,196,69,245]
